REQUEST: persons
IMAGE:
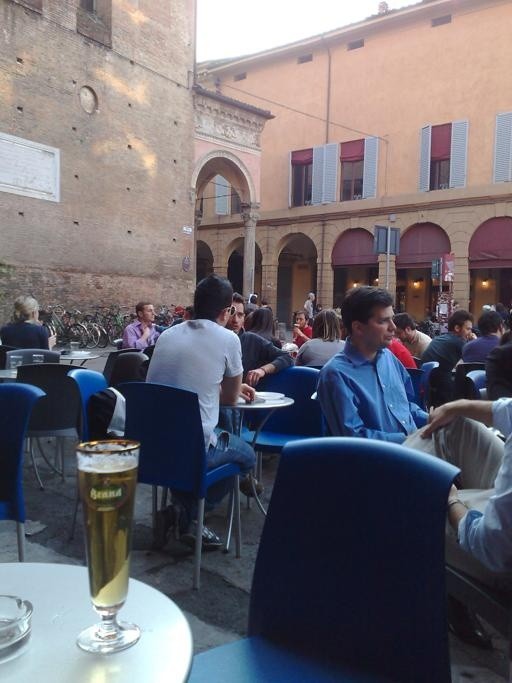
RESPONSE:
[0,295,57,350]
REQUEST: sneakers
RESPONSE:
[181,519,224,549]
[240,472,263,498]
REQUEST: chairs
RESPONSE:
[455,362,488,399]
[405,356,440,414]
[66,369,108,542]
[243,366,320,508]
[194,437,462,682]
[111,383,243,590]
[1,344,60,370]
[320,412,331,437]
[103,344,154,389]
[0,382,46,563]
[16,364,79,439]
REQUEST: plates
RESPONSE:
[255,391,285,400]
[72,351,91,354]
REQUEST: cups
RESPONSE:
[60,350,70,354]
[33,354,44,363]
[10,355,23,372]
[294,324,299,327]
[71,341,79,354]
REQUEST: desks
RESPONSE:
[218,392,295,554]
[61,351,103,367]
[1,562,194,682]
[2,371,63,492]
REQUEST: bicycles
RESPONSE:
[38,304,187,349]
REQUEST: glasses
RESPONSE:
[223,305,237,316]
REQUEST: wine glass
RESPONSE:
[74,437,141,654]
